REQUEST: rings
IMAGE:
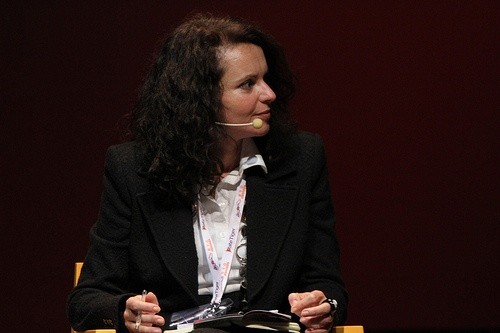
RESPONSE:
[321,299,337,314]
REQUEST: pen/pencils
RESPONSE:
[135,289,146,330]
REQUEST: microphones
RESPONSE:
[214,118,263,129]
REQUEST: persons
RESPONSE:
[67,18,349,333]
